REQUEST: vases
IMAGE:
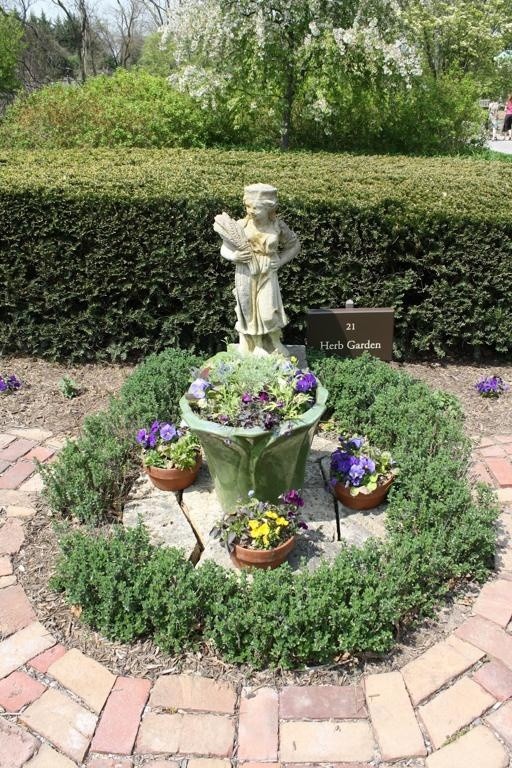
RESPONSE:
[222,531,295,570]
[143,455,203,490]
[330,473,395,510]
[177,375,330,516]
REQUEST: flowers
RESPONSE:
[210,486,310,549]
[138,418,203,472]
[324,434,399,496]
[186,347,318,435]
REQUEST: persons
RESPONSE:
[212,180,302,356]
[486,97,500,140]
[500,94,512,139]
